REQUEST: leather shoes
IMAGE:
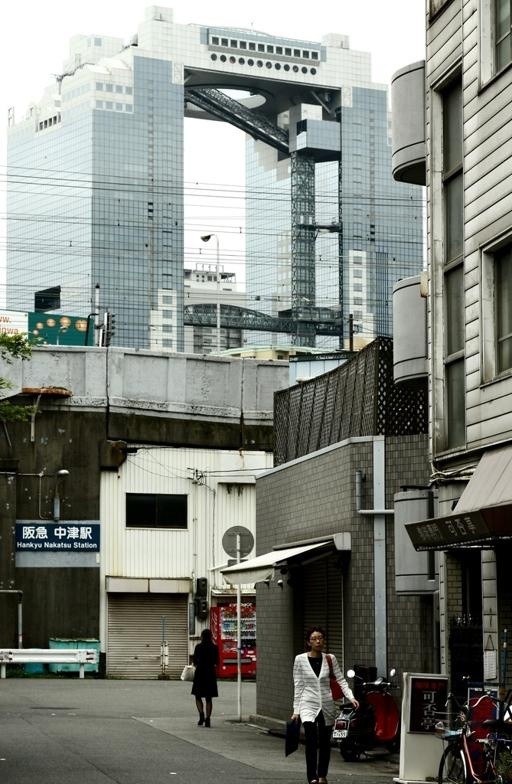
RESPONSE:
[310,779,318,784]
[318,777,328,784]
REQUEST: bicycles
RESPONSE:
[433,687,512,784]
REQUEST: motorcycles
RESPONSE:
[330,665,402,765]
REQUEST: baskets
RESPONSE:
[433,711,463,740]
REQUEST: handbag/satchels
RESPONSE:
[325,655,344,700]
[180,662,196,681]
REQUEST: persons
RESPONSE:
[190,628,220,728]
[290,627,363,783]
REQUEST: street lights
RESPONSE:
[200,233,221,357]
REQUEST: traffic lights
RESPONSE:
[102,332,112,348]
[102,312,117,334]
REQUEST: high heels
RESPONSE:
[204,717,210,727]
[198,712,204,725]
